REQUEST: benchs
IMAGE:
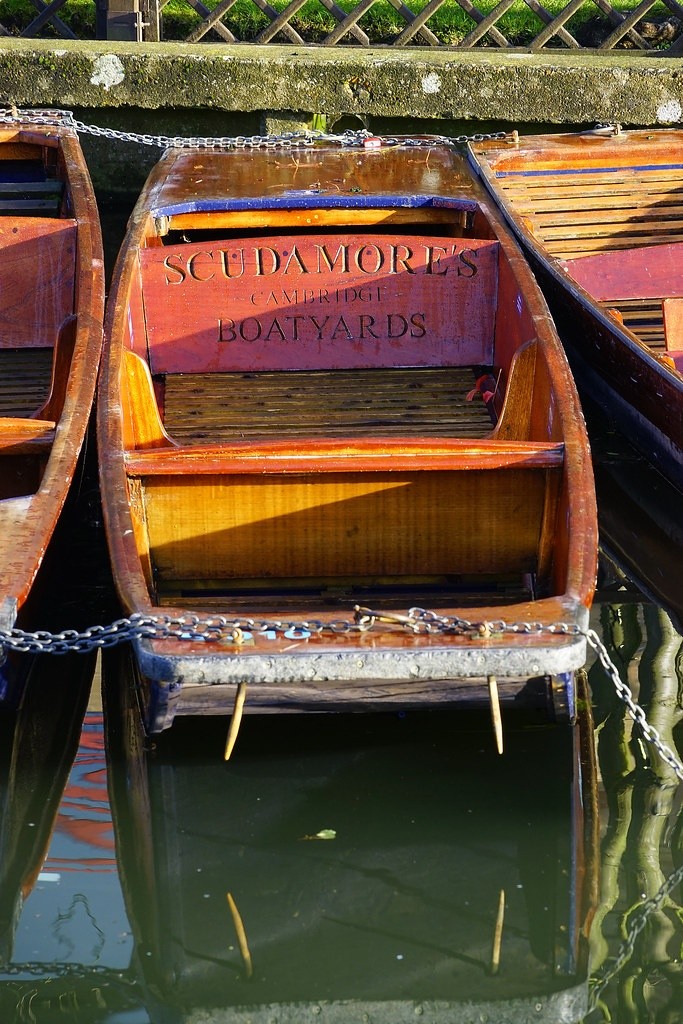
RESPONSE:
[140,234,503,436]
[0,417,57,498]
[0,217,78,416]
[123,434,564,588]
[495,146,683,375]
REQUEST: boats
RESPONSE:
[100,641,602,1023]
[0,108,105,663]
[95,135,600,717]
[462,128,683,527]
